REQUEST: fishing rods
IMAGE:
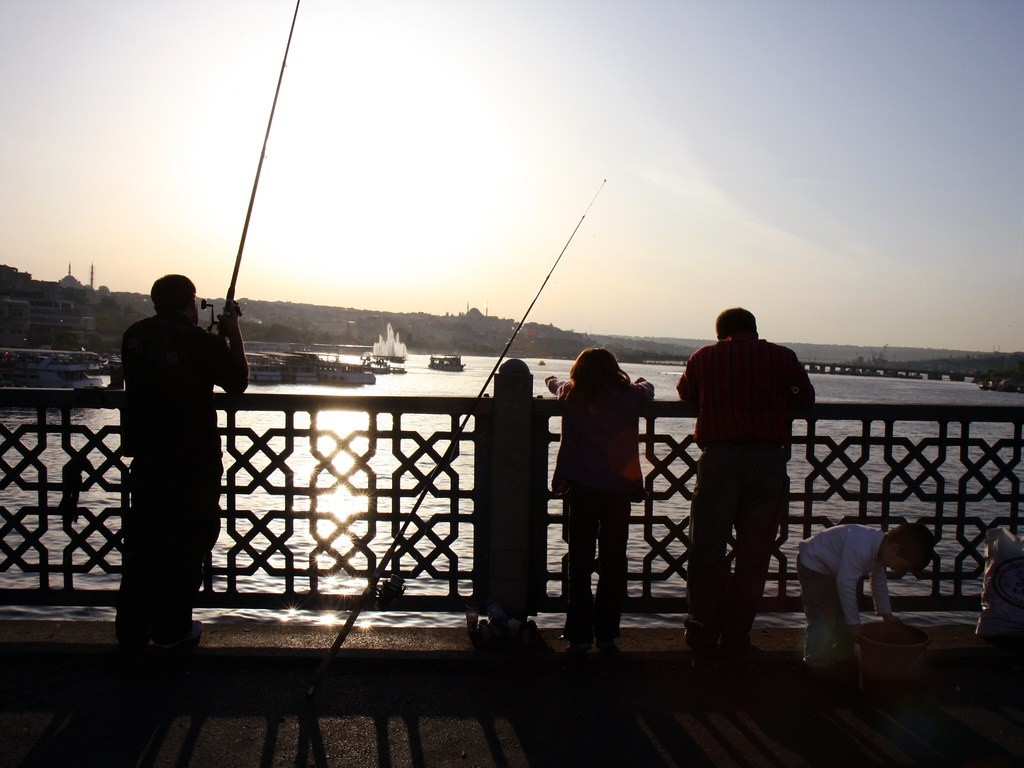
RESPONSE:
[299,177,608,711]
[199,0,302,333]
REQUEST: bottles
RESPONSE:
[382,571,406,598]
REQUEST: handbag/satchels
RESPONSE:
[974,528,1024,636]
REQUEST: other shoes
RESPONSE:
[683,629,717,649]
[567,641,592,652]
[156,620,203,648]
[595,639,613,649]
[719,632,751,656]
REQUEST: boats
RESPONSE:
[0,347,105,388]
[245,350,392,385]
[979,378,1024,393]
[427,354,466,372]
[539,360,546,365]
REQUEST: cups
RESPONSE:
[108,359,124,389]
[465,610,480,632]
[506,621,521,640]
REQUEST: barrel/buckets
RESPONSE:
[854,621,932,705]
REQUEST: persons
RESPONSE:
[677,309,815,678]
[115,275,249,647]
[545,348,655,652]
[796,523,935,672]
